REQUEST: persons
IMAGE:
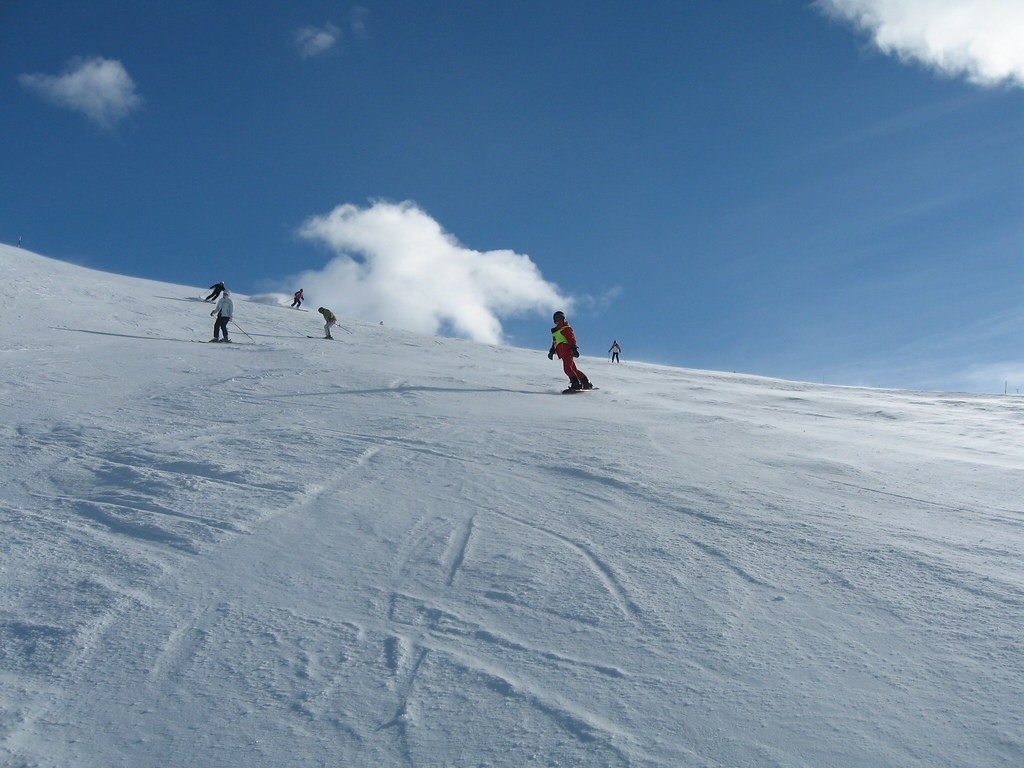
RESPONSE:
[548,311,593,391]
[318,307,337,339]
[290,289,304,308]
[608,340,621,363]
[209,289,233,342]
[205,281,225,302]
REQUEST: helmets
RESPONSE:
[553,311,566,321]
[223,289,231,296]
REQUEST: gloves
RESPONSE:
[548,352,553,359]
[210,311,214,317]
[573,347,579,357]
[229,317,232,322]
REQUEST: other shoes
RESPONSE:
[582,380,594,389]
[210,337,219,341]
[569,383,581,390]
[221,337,228,340]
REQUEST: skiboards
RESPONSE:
[190,339,232,343]
[289,307,308,312]
[306,335,334,340]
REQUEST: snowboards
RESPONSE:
[562,387,600,394]
